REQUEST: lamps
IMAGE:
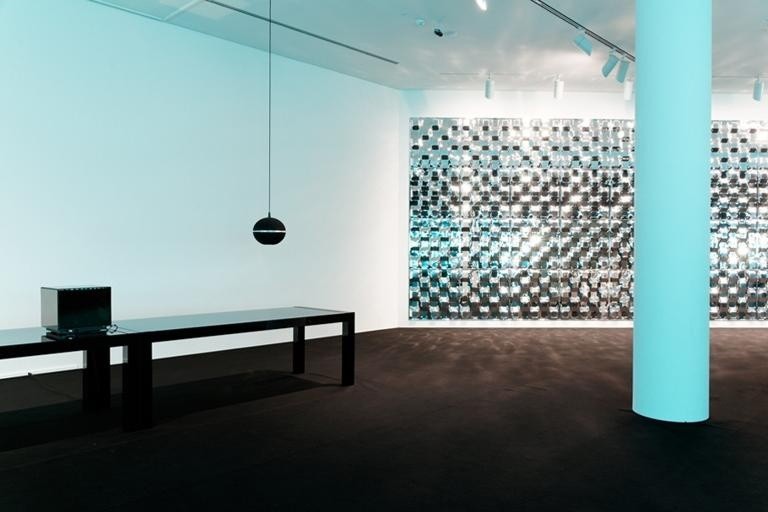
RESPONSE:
[532,0,635,82]
[252,0,286,245]
[441,72,767,101]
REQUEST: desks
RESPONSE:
[0,322,126,426]
[109,308,356,414]
